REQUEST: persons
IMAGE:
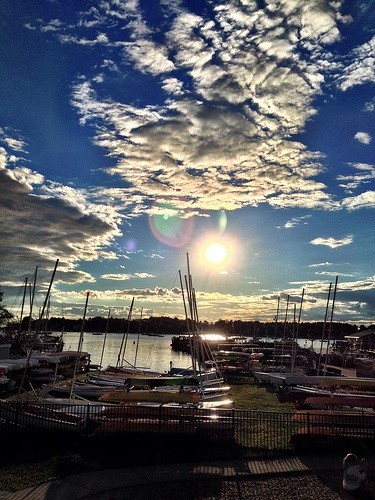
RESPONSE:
[179,384,184,394]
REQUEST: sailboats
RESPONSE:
[1,254,375,448]
